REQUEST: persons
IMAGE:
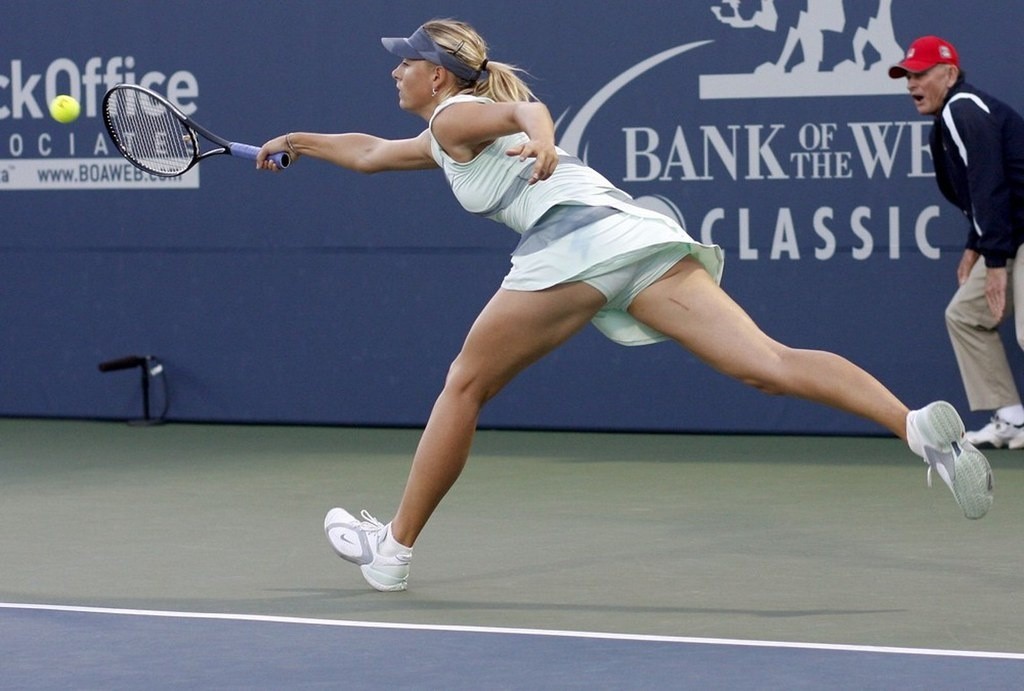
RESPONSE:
[885,35,1024,448]
[252,17,999,596]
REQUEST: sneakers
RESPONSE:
[906,399,993,520]
[965,413,1024,450]
[322,507,412,593]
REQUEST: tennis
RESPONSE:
[49,94,81,124]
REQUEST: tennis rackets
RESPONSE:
[102,83,291,179]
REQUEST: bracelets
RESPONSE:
[286,132,302,157]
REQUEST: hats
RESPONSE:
[381,27,490,83]
[888,34,960,80]
